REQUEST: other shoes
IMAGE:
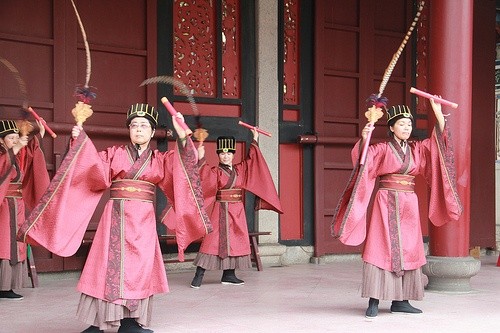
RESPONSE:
[0,289,24,300]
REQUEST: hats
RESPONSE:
[126,102,160,130]
[216,135,236,155]
[0,120,20,138]
[386,105,414,129]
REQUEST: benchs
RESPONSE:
[80,230,272,273]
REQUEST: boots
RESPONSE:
[221,269,244,285]
[365,297,380,319]
[117,318,154,333]
[80,325,104,333]
[391,300,423,314]
[190,266,206,288]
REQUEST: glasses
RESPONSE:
[129,123,150,129]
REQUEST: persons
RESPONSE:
[67,104,195,333]
[191,127,259,289]
[0,117,48,300]
[330,95,448,318]
